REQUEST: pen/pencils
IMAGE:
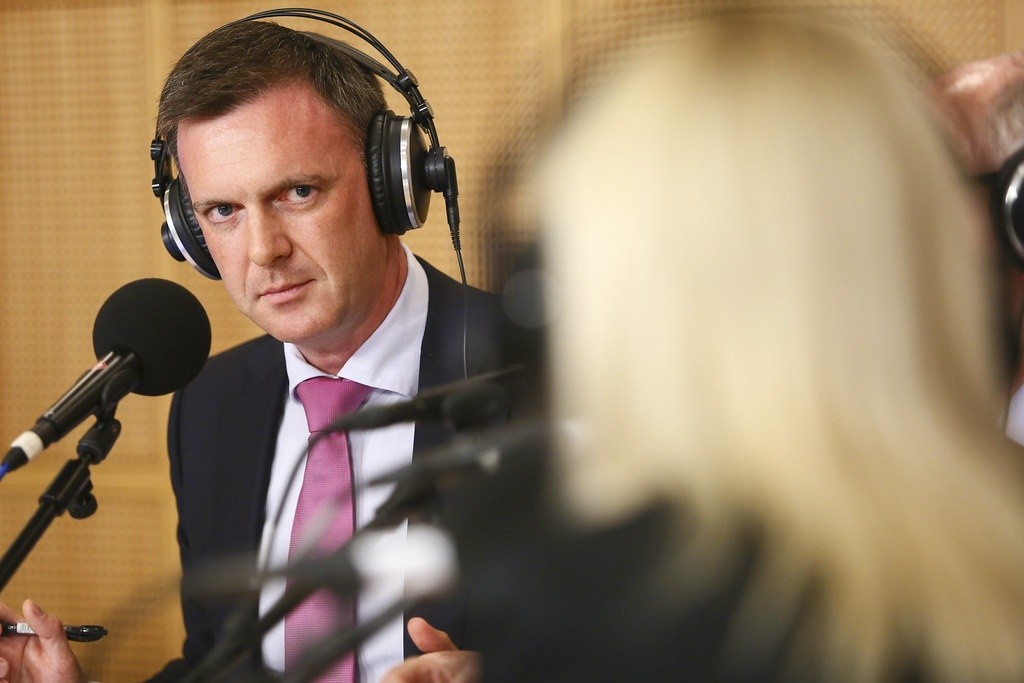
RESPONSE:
[0,621,109,643]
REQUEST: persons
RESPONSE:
[0,22,523,683]
[523,18,1024,683]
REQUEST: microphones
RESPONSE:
[4,277,212,471]
[320,363,554,486]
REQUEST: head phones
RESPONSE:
[150,6,461,280]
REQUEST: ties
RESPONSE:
[285,376,373,683]
